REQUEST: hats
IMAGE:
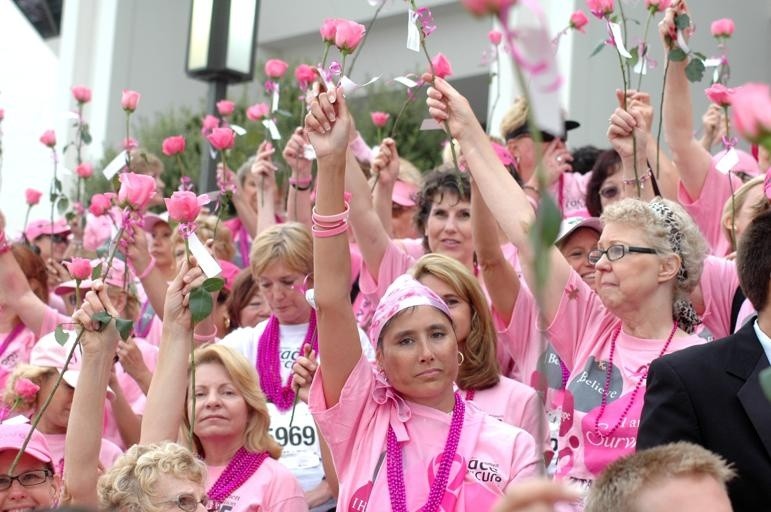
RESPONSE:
[55,256,137,300]
[143,210,169,233]
[30,329,84,389]
[216,258,242,296]
[368,96,605,245]
[26,219,72,243]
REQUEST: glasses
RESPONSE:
[216,290,229,303]
[70,288,91,305]
[0,469,52,491]
[152,493,210,512]
[46,234,68,245]
[587,243,663,263]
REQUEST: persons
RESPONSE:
[1,0,770,511]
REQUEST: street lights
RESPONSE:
[178,0,260,194]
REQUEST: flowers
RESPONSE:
[0,1,771,476]
